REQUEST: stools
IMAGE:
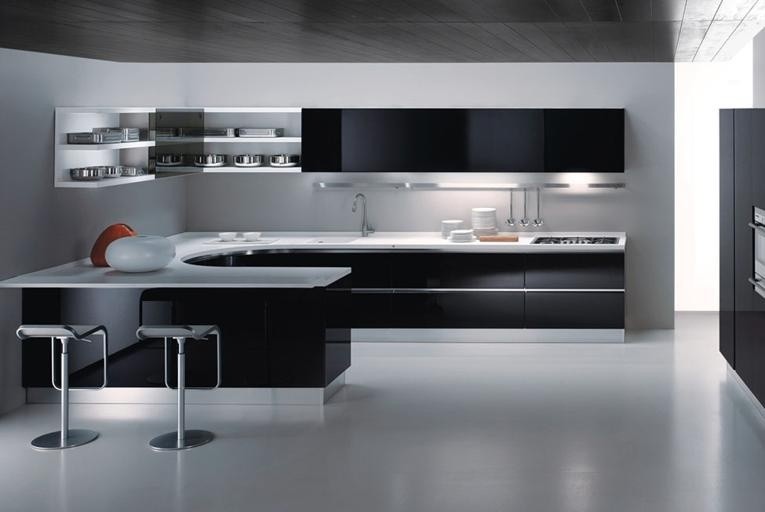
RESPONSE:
[135,324,219,452]
[16,323,107,451]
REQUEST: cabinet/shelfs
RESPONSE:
[22,254,625,403]
[155,108,204,180]
[204,107,301,172]
[302,108,631,173]
[56,107,155,188]
[720,109,765,412]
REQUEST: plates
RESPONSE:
[441,207,498,244]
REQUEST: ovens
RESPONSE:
[747,206,765,298]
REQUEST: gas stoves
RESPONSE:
[531,236,619,245]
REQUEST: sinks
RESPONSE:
[278,235,356,244]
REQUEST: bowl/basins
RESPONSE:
[69,164,136,180]
[156,151,300,168]
[218,231,237,242]
[243,232,262,240]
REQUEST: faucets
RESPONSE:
[351,193,375,237]
